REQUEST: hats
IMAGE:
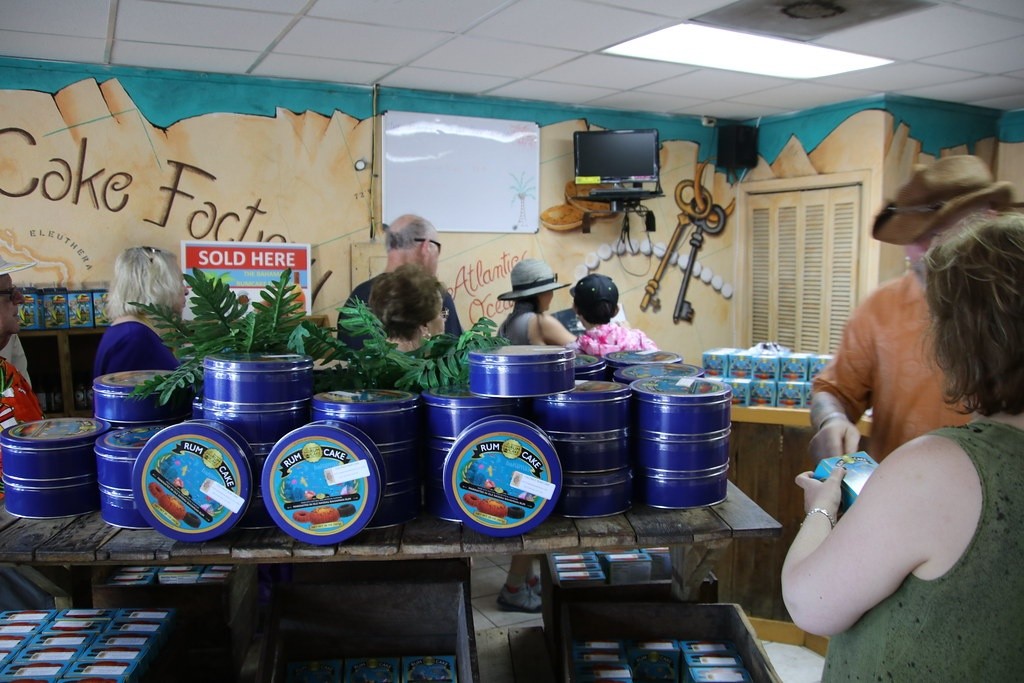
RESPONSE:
[497,259,573,301]
[872,154,1014,246]
[569,273,619,310]
[0,254,37,277]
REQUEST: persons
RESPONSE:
[560,273,658,355]
[93,246,190,377]
[806,154,1015,464]
[495,258,577,614]
[367,264,449,358]
[334,214,464,351]
[0,256,46,500]
[780,218,1024,683]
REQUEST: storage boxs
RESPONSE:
[541,545,782,682]
[812,451,879,511]
[14,287,110,329]
[702,347,835,409]
[0,558,482,683]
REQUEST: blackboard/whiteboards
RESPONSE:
[380,110,540,233]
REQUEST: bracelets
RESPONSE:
[807,508,835,528]
[819,412,847,428]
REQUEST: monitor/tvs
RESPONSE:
[574,128,660,194]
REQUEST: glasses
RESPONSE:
[0,286,17,302]
[142,244,160,262]
[413,237,444,257]
[436,306,449,322]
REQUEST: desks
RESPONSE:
[16,323,111,414]
[1,478,783,609]
[719,405,874,658]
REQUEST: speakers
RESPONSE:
[718,124,760,168]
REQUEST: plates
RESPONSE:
[539,180,618,230]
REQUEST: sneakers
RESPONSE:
[498,586,544,613]
[528,575,541,595]
